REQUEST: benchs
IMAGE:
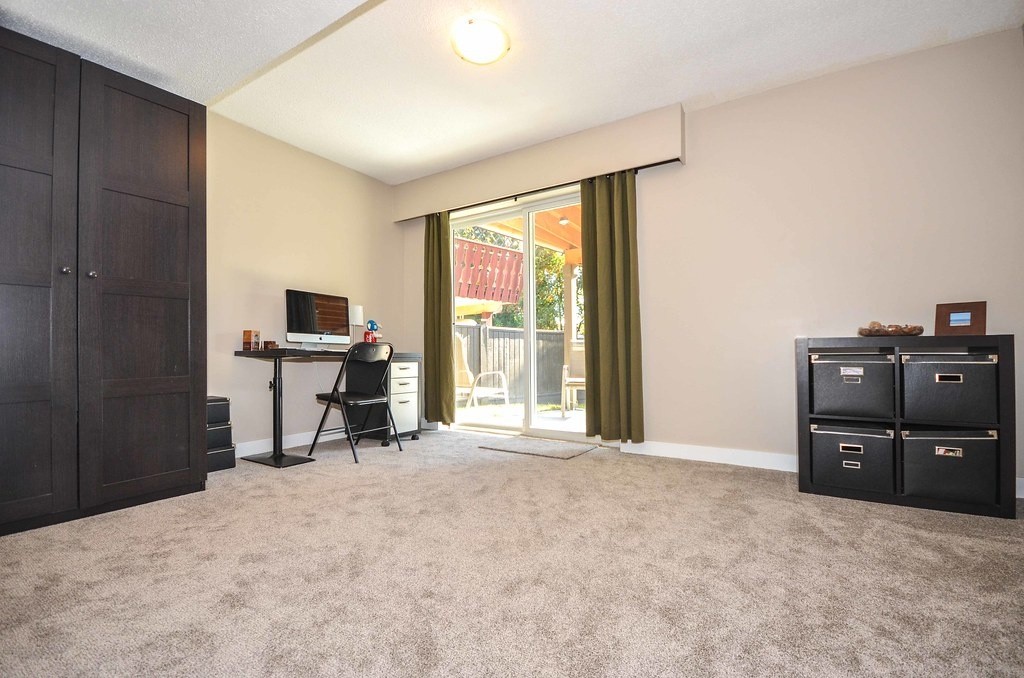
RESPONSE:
[560,364,585,420]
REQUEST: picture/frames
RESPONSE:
[935,301,986,335]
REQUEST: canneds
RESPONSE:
[364,331,377,343]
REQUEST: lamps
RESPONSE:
[558,217,570,226]
[348,304,364,345]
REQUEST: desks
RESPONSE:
[234,347,423,469]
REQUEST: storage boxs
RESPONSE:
[243,330,260,351]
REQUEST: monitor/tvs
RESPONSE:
[285,289,351,351]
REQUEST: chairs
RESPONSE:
[308,342,403,464]
[455,333,508,416]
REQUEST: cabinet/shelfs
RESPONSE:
[0,25,208,537]
[794,333,1017,519]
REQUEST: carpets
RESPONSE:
[478,435,600,460]
